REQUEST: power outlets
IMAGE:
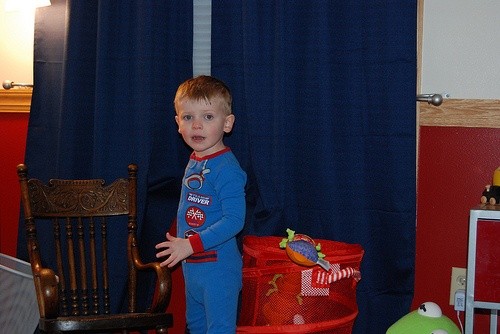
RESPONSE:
[449,267,467,305]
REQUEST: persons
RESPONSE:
[155,75,247,334]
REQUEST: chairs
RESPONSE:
[15,161,172,334]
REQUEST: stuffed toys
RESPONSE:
[386,302,461,334]
[279,228,325,267]
[481,167,500,205]
